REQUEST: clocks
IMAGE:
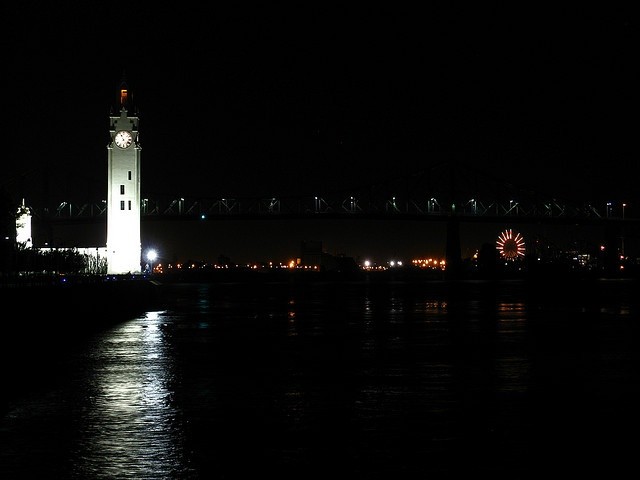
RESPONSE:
[114,130,133,149]
